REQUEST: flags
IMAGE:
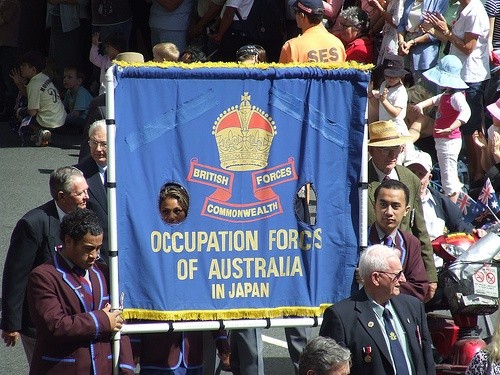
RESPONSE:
[474,178,500,222]
[457,191,482,224]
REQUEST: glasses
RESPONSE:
[371,269,405,282]
[340,22,356,30]
[377,146,404,154]
[87,141,108,150]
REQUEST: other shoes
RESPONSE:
[31,129,51,147]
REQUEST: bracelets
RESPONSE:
[369,94,374,99]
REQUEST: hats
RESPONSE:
[402,150,432,172]
[376,55,410,77]
[486,98,500,120]
[422,54,469,89]
[292,0,325,16]
[368,120,413,147]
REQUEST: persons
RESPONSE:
[300,336,352,375]
[465,309,500,375]
[355,179,431,303]
[2,165,110,347]
[405,86,438,168]
[0,0,500,177]
[78,119,108,257]
[319,243,435,375]
[233,313,313,375]
[474,98,500,195]
[138,318,231,375]
[402,150,488,309]
[366,121,438,299]
[412,55,471,204]
[369,54,415,166]
[27,207,135,375]
[160,187,189,224]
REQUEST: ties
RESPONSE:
[382,308,409,375]
[73,266,93,309]
[103,169,108,192]
[382,235,394,247]
[384,176,391,181]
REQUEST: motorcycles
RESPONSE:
[419,231,500,375]
